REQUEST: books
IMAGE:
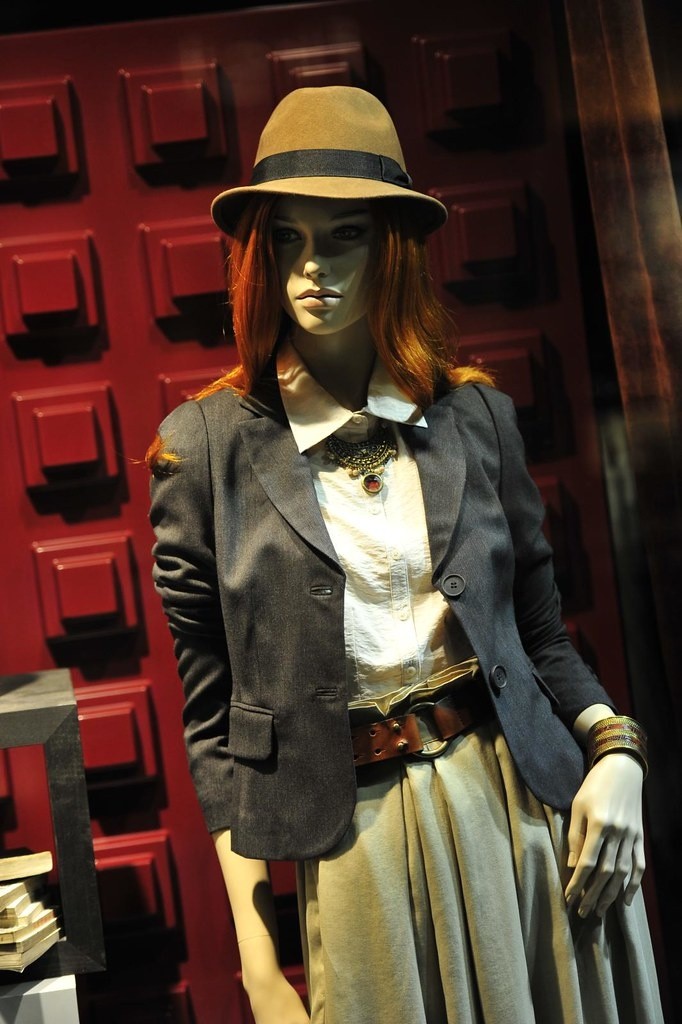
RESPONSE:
[0,851,65,973]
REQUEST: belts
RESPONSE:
[350,677,494,767]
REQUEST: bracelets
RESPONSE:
[583,715,652,780]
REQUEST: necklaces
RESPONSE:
[321,419,398,493]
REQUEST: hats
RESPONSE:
[210,87,446,238]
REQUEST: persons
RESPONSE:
[147,82,666,1024]
